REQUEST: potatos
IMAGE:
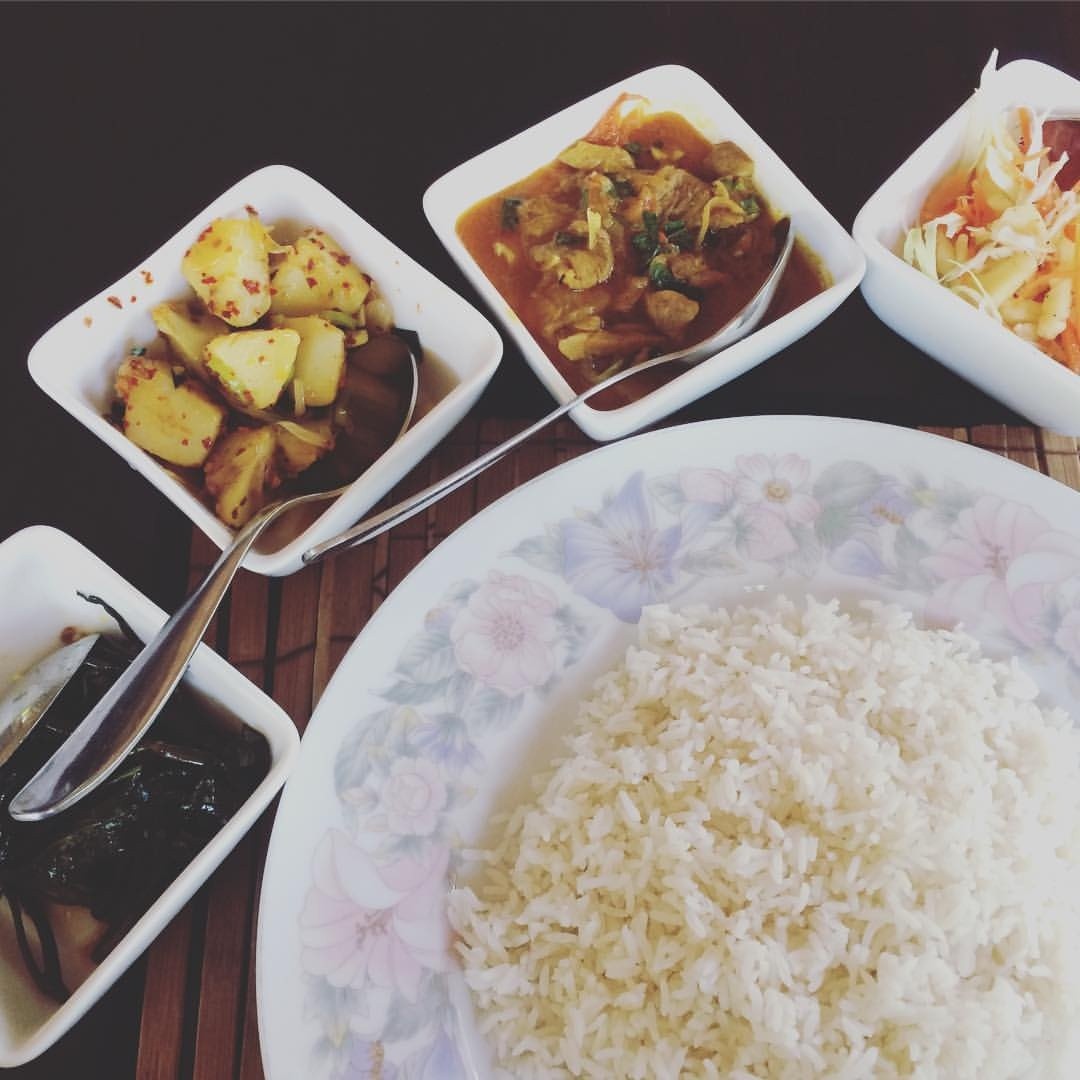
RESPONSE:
[113,204,393,530]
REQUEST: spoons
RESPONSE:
[2,630,100,768]
[4,332,415,818]
[294,213,796,560]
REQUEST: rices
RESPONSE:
[443,595,1053,1080]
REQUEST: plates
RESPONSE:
[254,414,1080,1080]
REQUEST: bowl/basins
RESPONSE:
[1,525,301,1068]
[851,56,1078,439]
[26,165,506,582]
[419,64,866,445]
